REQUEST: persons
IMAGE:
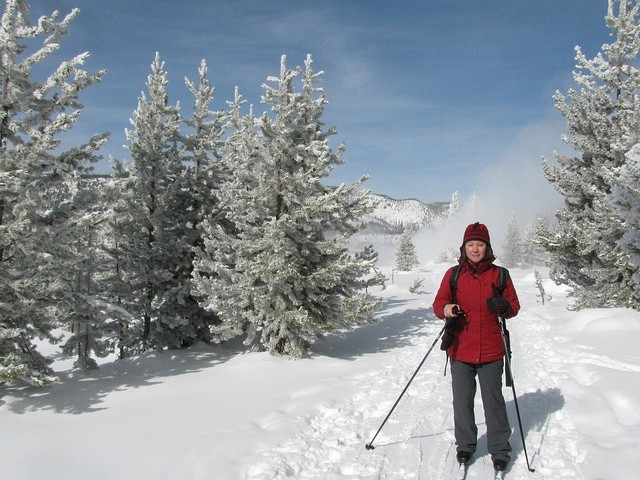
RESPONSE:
[432,221,521,471]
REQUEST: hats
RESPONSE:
[463,222,490,244]
[488,289,511,318]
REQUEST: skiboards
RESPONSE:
[454,463,506,480]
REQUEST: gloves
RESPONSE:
[440,307,465,350]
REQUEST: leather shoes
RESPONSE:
[494,459,507,470]
[457,451,471,462]
